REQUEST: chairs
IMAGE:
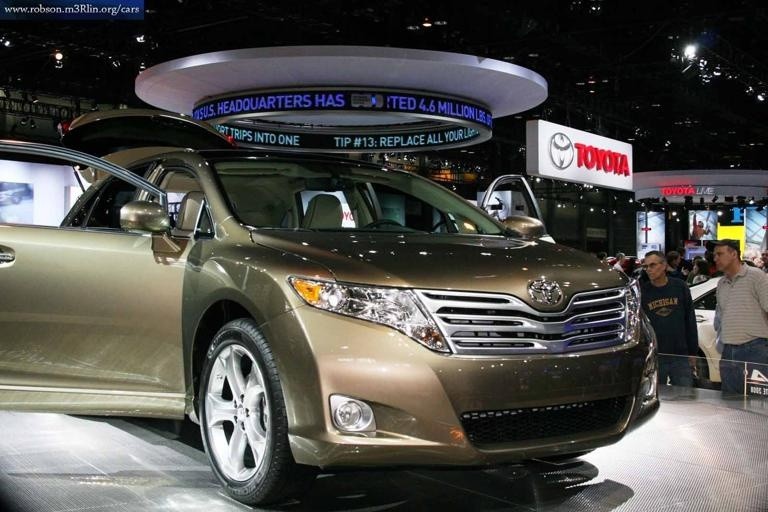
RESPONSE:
[297,193,346,231]
[171,190,207,239]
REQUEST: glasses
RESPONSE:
[642,262,659,270]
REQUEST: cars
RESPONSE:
[0,182,30,206]
[687,272,722,387]
[0,107,659,506]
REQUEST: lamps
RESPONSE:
[627,189,767,220]
[0,22,157,132]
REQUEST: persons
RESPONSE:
[589,250,767,289]
[715,238,768,393]
[692,212,710,239]
[714,260,756,353]
[640,251,699,387]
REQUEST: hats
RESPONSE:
[706,239,741,253]
